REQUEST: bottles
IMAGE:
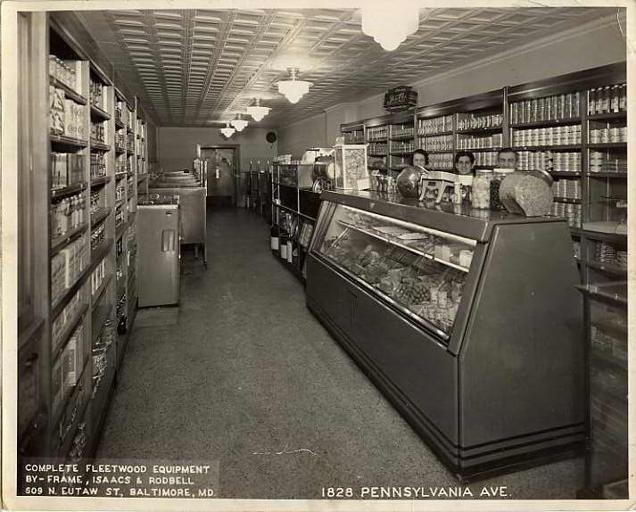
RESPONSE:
[472,169,494,210]
[490,167,517,211]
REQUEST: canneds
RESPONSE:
[49,54,76,91]
[512,124,627,173]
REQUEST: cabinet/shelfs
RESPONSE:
[338,58,627,288]
[41,20,152,484]
[267,182,587,482]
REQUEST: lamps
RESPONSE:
[217,67,317,141]
[361,3,420,55]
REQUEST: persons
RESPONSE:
[454,150,476,176]
[411,148,430,171]
[497,147,521,172]
